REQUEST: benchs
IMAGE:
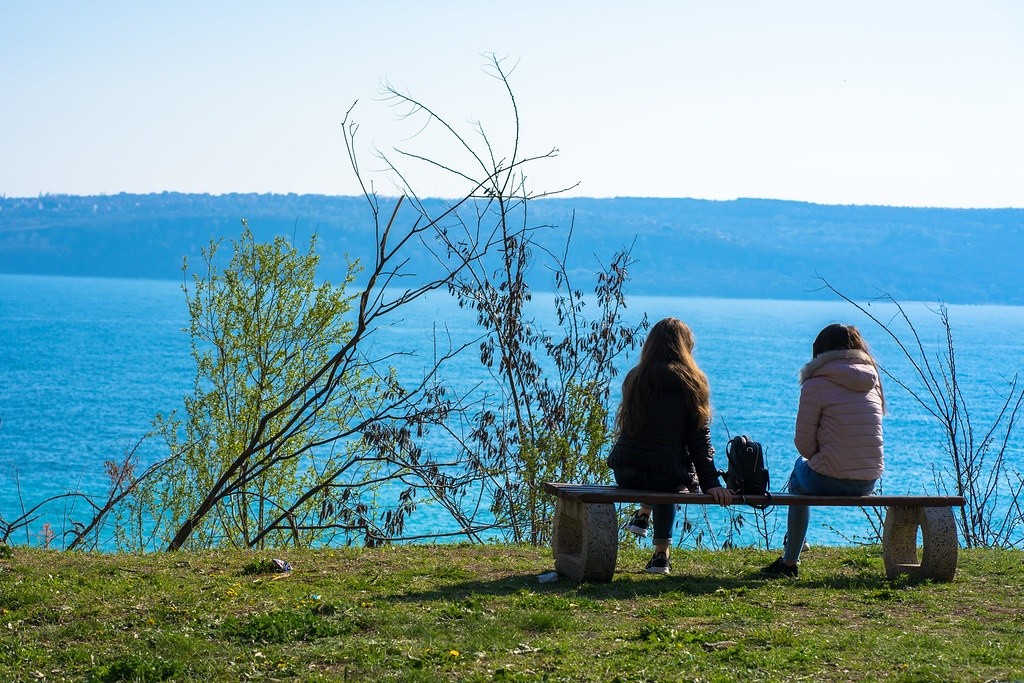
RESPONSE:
[542,482,966,585]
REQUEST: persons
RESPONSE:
[759,323,887,579]
[607,317,733,576]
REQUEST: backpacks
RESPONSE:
[717,434,771,509]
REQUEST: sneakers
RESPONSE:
[760,556,798,578]
[625,510,650,538]
[644,549,671,574]
[783,532,811,552]
